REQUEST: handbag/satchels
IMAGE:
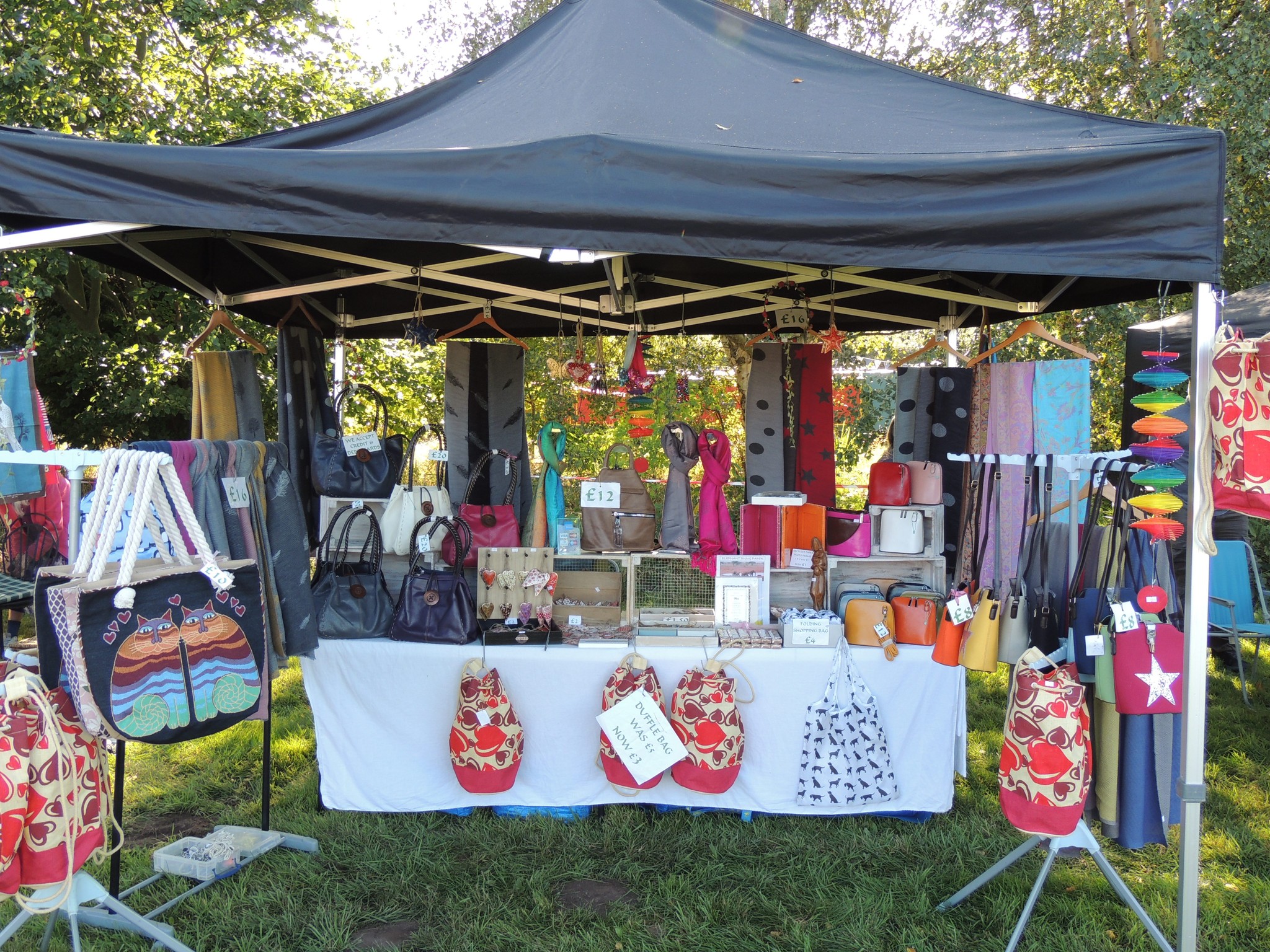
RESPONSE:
[596,653,667,797]
[835,578,947,661]
[998,646,1092,837]
[449,658,524,795]
[1197,324,1270,556]
[35,449,267,745]
[0,668,121,912]
[1066,589,1184,714]
[932,577,1063,674]
[581,444,655,551]
[796,637,901,805]
[670,639,755,795]
[825,461,943,557]
[311,384,520,646]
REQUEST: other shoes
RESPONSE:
[3,632,18,652]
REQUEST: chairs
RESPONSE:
[2,513,60,585]
[1208,541,1270,709]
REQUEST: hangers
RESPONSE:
[965,313,1099,370]
[184,293,268,359]
[892,318,970,369]
[744,297,820,349]
[276,281,325,335]
[436,313,529,350]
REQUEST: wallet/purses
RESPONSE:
[739,502,826,569]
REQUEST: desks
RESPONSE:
[0,573,35,662]
[299,638,969,817]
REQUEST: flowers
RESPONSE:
[761,281,814,449]
[324,341,361,385]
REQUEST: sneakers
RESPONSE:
[1211,648,1248,673]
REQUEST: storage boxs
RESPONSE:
[778,617,844,648]
[316,494,478,609]
[751,493,807,506]
[550,571,623,628]
[476,618,562,646]
[769,504,947,625]
[635,608,719,648]
[153,836,242,881]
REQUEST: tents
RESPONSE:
[1,0,1224,950]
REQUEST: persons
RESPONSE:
[809,537,827,612]
